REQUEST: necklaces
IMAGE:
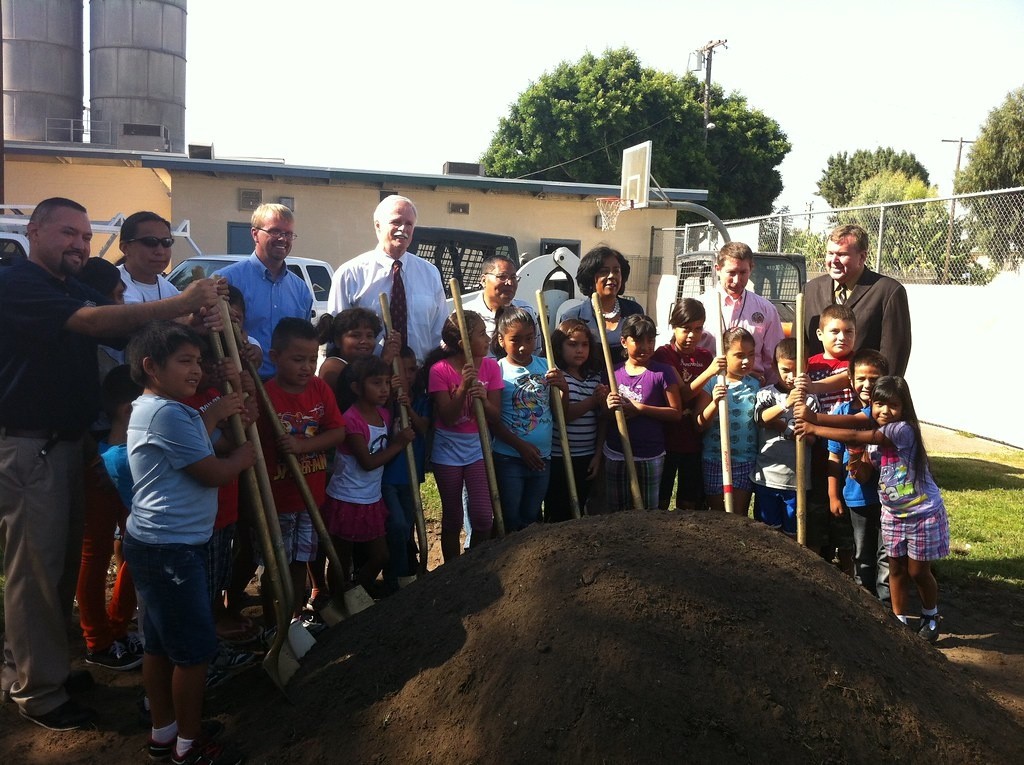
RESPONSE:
[602,298,620,319]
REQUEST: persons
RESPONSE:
[207,203,313,383]
[792,375,950,644]
[695,328,761,516]
[447,255,544,361]
[316,308,383,390]
[99,364,260,692]
[790,224,912,378]
[685,242,786,387]
[748,337,821,542]
[229,286,263,371]
[175,341,255,702]
[555,247,644,373]
[121,318,258,765]
[328,299,727,608]
[828,349,892,607]
[326,194,449,369]
[0,197,230,731]
[793,304,856,572]
[255,316,346,621]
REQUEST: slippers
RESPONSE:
[214,613,262,644]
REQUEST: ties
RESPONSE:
[390,259,408,347]
[834,283,847,304]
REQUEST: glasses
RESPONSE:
[120,236,174,247]
[255,226,298,241]
[484,272,522,282]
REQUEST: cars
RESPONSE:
[165,252,335,331]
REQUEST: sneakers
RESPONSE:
[204,641,260,689]
[148,720,224,760]
[86,632,144,670]
[171,735,244,765]
[261,613,326,651]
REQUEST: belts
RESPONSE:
[0,426,86,439]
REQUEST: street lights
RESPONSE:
[942,135,981,285]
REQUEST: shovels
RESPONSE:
[191,266,299,701]
[223,298,374,630]
[378,292,430,590]
[209,273,319,657]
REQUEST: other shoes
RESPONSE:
[139,703,152,729]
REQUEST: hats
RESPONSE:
[77,257,121,295]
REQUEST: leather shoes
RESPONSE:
[19,701,97,731]
[0,671,94,702]
[917,610,941,644]
